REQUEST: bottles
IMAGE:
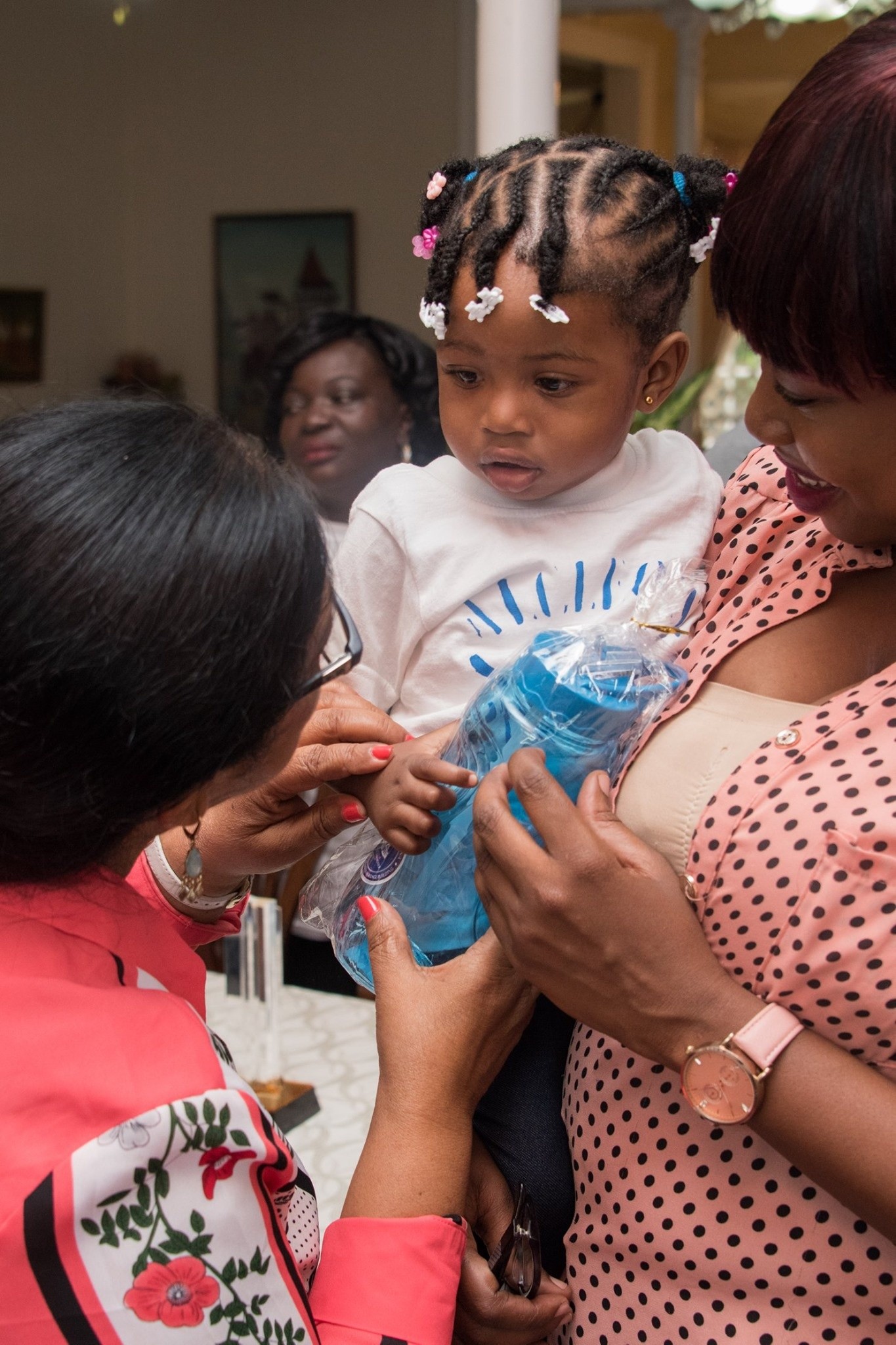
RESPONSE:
[326,630,685,995]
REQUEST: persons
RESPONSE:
[263,307,455,564]
[0,7,896,1345]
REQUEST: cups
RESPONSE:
[224,900,285,1097]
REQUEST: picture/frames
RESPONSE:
[213,210,358,437]
[0,289,44,384]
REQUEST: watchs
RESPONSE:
[678,1000,804,1126]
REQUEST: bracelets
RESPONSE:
[144,833,254,911]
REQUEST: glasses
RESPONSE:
[287,587,363,700]
